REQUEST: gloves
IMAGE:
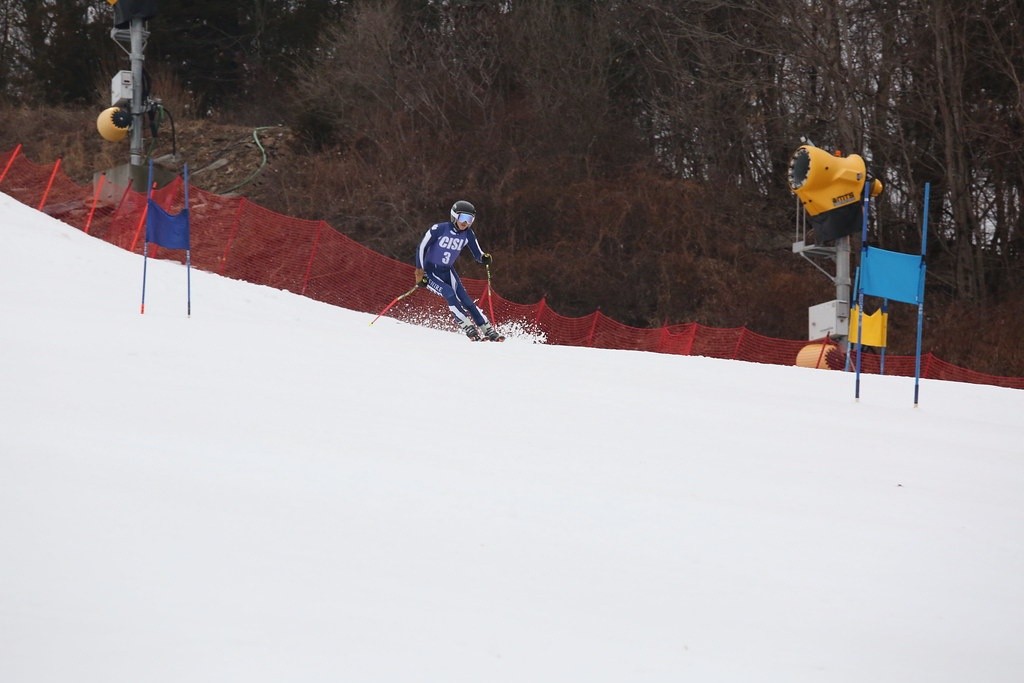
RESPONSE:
[418,277,429,287]
[482,254,492,267]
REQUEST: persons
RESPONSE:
[415,201,505,342]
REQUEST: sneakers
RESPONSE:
[485,327,505,343]
[463,325,481,342]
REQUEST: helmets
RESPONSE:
[450,200,477,232]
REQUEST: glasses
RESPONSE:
[457,213,475,224]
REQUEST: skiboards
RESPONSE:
[480,335,506,342]
